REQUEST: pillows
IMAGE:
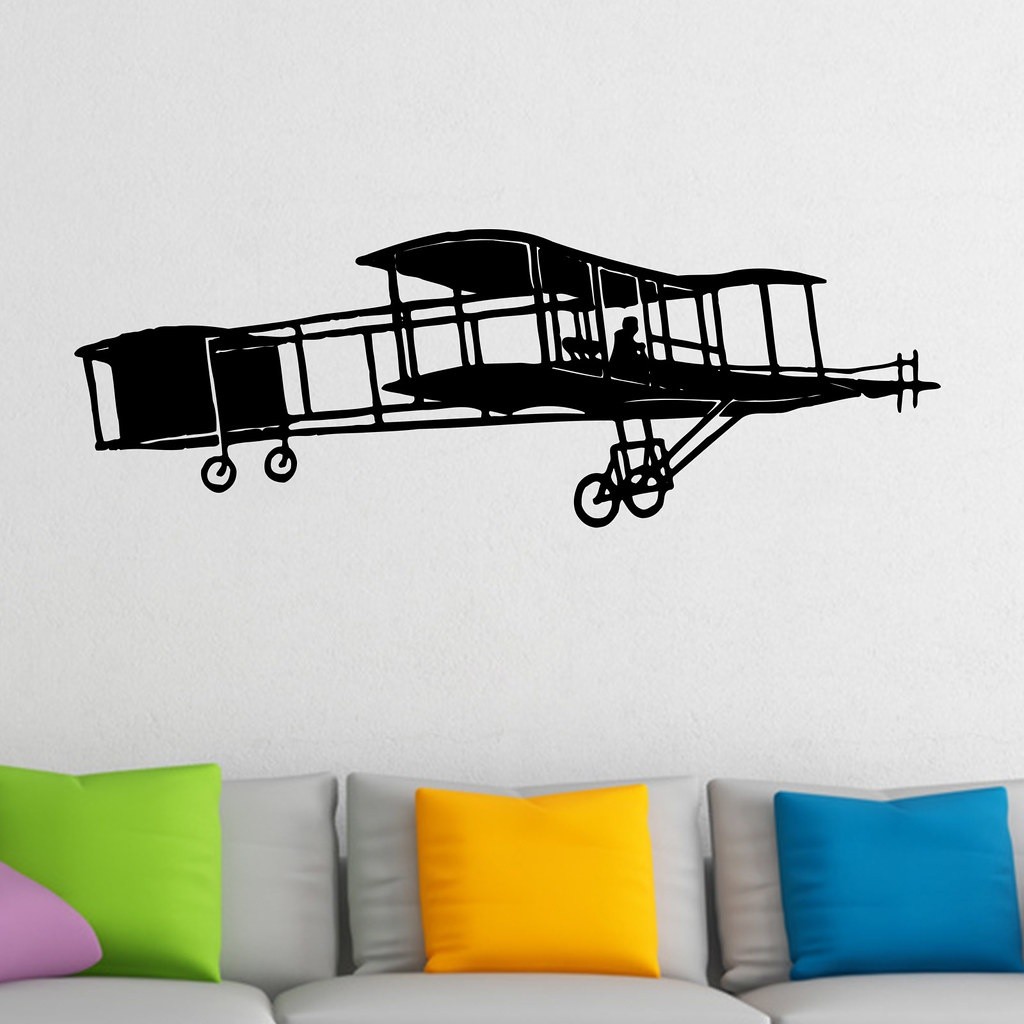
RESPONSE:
[706,776,1024,992]
[0,859,101,983]
[414,781,661,978]
[775,785,1024,980]
[0,763,226,983]
[346,772,714,988]
[222,773,343,999]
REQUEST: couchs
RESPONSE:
[0,853,1024,1024]
